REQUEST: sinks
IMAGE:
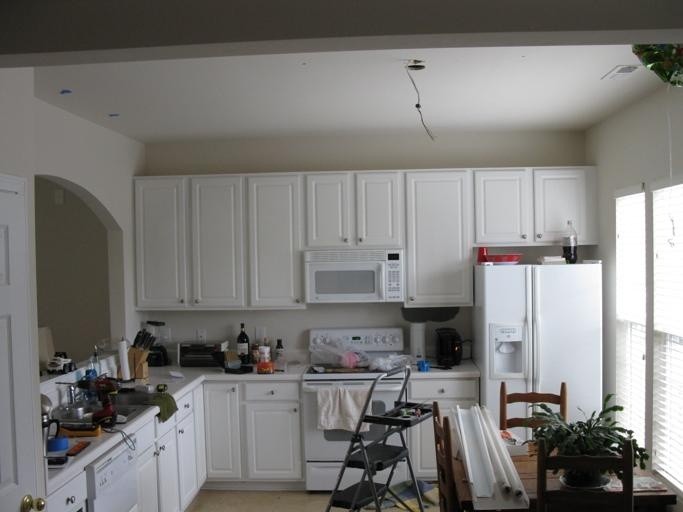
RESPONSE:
[62,403,148,421]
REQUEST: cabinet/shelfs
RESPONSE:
[43,461,88,512]
[151,372,197,510]
[137,405,182,511]
[244,351,307,494]
[473,169,600,247]
[206,363,243,492]
[411,359,480,488]
[132,175,243,307]
[406,169,473,308]
[244,172,306,310]
[325,365,434,512]
[1,175,40,511]
[307,170,405,249]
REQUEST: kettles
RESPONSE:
[40,394,60,456]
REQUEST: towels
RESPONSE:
[143,392,178,423]
[316,384,373,433]
[363,479,440,512]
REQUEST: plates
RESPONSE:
[486,261,518,265]
[483,254,524,261]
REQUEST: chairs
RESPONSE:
[500,382,568,441]
[434,402,451,512]
[533,440,634,512]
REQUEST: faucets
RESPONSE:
[55,382,76,404]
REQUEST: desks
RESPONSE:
[453,439,676,512]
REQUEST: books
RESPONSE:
[603,476,668,493]
[538,255,566,264]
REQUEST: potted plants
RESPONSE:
[525,392,649,488]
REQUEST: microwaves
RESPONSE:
[303,250,407,303]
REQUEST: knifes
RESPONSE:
[129,328,156,362]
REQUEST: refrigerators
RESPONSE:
[474,264,603,446]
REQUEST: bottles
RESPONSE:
[237,323,250,364]
[276,339,284,359]
[562,220,578,263]
